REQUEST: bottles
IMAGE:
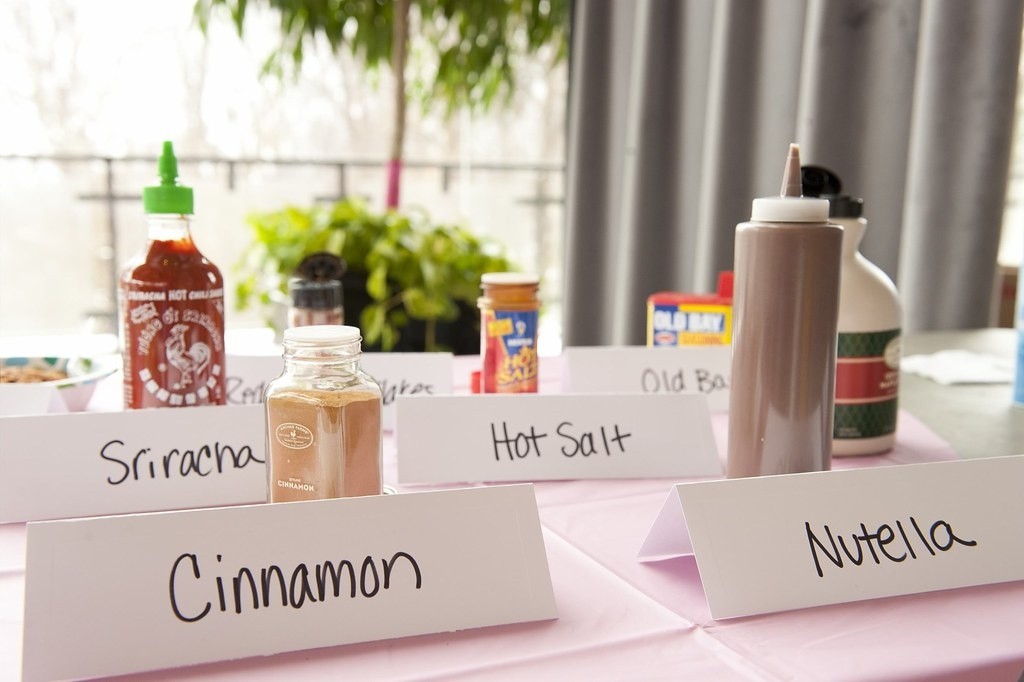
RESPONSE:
[796,164,904,459]
[646,271,732,347]
[724,143,844,477]
[119,141,228,410]
[264,324,385,506]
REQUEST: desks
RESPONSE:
[0,324,1022,682]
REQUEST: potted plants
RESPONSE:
[195,0,580,357]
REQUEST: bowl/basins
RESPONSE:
[1,353,118,419]
